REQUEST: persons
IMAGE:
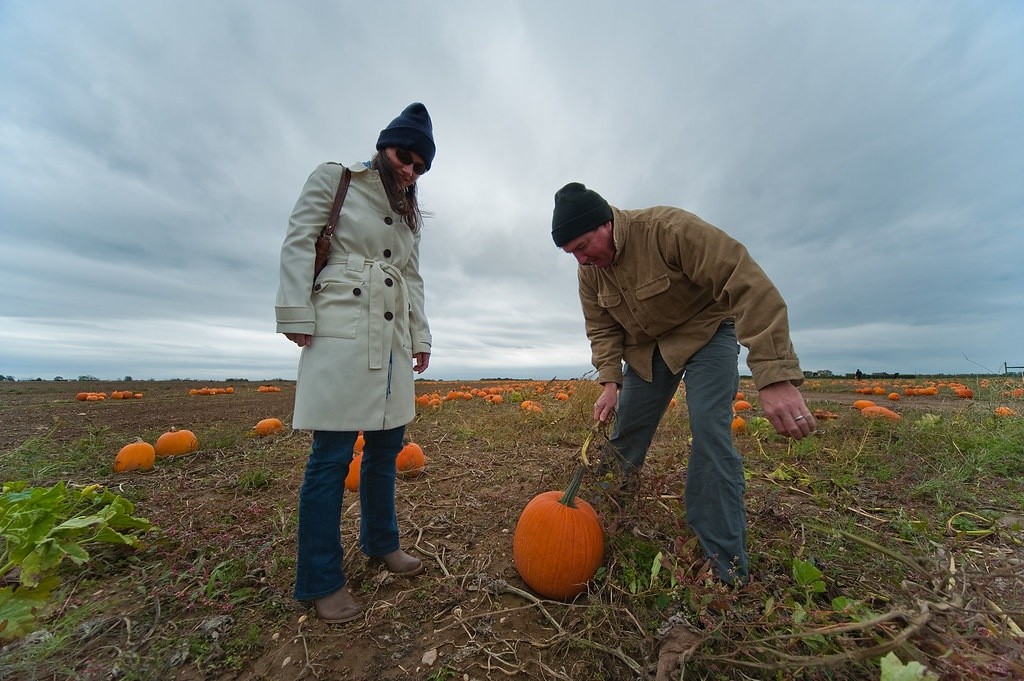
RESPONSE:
[275,103,436,624]
[550,183,818,589]
[855,369,862,381]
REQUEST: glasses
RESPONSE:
[395,145,426,176]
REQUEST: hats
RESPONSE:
[551,183,613,247]
[376,102,436,172]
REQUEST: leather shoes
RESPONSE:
[382,548,423,576]
[314,585,365,625]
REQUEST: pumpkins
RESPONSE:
[513,491,604,600]
[343,379,752,492]
[114,440,155,472]
[77,390,143,401]
[189,386,233,395]
[155,426,198,456]
[795,379,1024,421]
[259,386,281,392]
[255,418,282,436]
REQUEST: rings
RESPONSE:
[795,415,804,422]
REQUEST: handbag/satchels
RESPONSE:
[314,166,351,277]
